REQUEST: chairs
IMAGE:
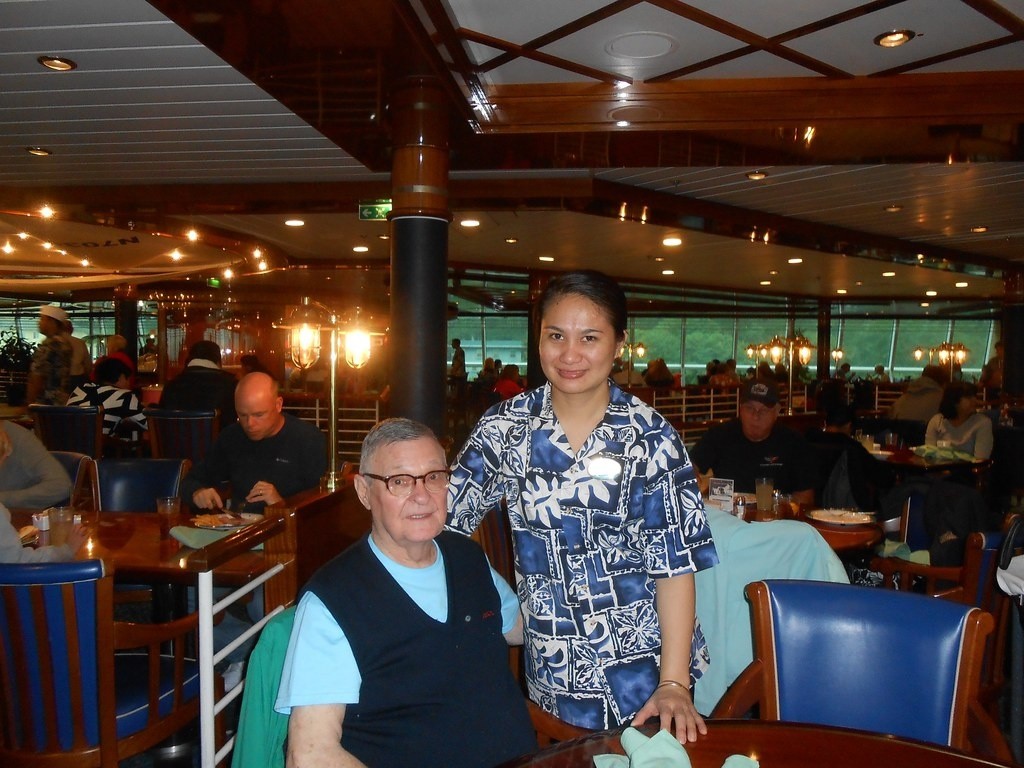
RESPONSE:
[0,402,1024,768]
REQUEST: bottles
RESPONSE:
[735,495,746,521]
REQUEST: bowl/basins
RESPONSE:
[867,451,895,460]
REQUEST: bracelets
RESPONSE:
[654,681,690,693]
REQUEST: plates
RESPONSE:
[733,492,756,503]
[195,513,264,530]
[721,501,733,512]
[805,508,878,526]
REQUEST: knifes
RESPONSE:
[212,501,244,521]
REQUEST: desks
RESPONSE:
[735,502,885,548]
[5,506,265,763]
[864,446,991,482]
[495,717,1019,767]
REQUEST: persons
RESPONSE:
[242,355,273,377]
[27,305,93,404]
[67,357,159,456]
[688,379,814,505]
[0,420,72,509]
[924,382,994,462]
[180,374,329,690]
[889,366,950,427]
[813,409,882,513]
[161,343,239,428]
[610,357,890,390]
[479,357,529,402]
[446,274,719,745]
[449,338,465,397]
[89,335,135,386]
[0,431,94,565]
[979,341,1003,388]
[143,337,157,355]
[274,418,527,768]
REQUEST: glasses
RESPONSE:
[361,466,453,496]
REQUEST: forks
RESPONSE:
[237,499,247,515]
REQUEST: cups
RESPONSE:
[861,434,874,451]
[884,433,897,450]
[48,505,72,545]
[937,440,952,447]
[755,477,774,511]
[156,497,181,536]
[772,488,803,521]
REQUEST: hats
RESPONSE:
[38,306,66,323]
[740,378,779,408]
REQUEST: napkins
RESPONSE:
[721,754,760,768]
[169,524,265,551]
[592,726,691,767]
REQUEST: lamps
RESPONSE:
[913,341,970,381]
[273,296,390,489]
[747,330,815,417]
